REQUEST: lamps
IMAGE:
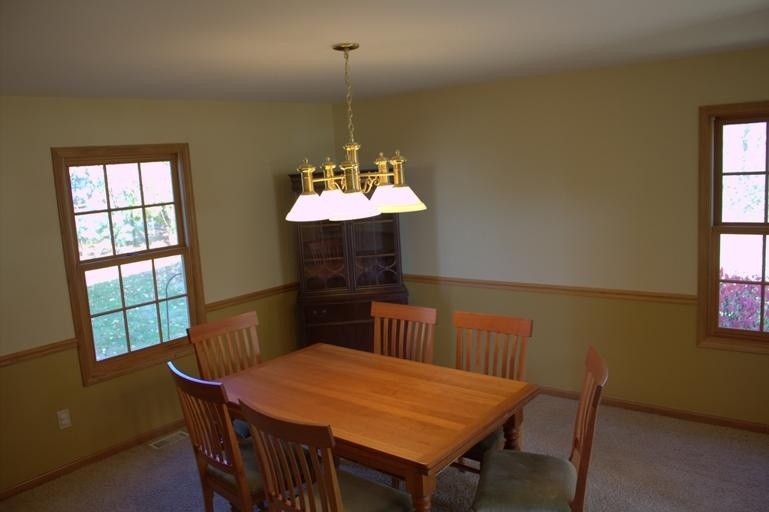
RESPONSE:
[285,42,430,225]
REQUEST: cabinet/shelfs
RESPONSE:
[289,170,409,353]
